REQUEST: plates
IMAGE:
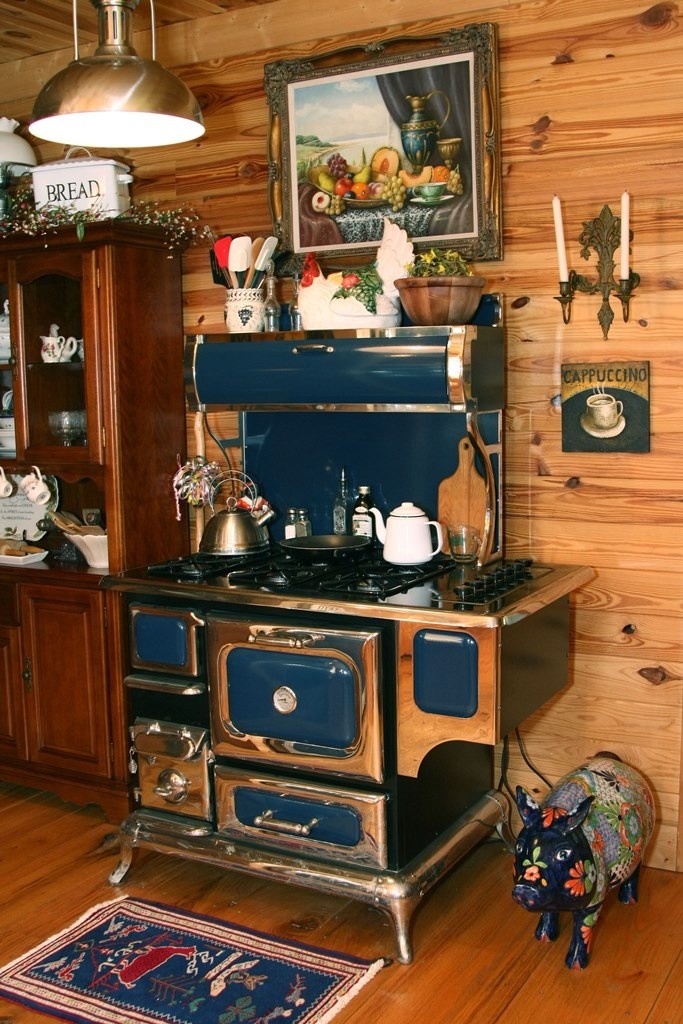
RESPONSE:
[0,551,49,566]
[579,411,626,439]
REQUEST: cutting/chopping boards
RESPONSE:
[438,440,491,554]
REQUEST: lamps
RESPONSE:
[28,0,206,148]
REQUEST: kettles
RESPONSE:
[197,469,277,557]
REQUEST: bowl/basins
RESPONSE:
[0,417,15,450]
[61,532,109,569]
[393,278,486,325]
[48,410,87,447]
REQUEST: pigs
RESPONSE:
[511,751,656,969]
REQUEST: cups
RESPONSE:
[586,394,623,429]
[447,523,482,563]
[20,465,51,506]
[0,467,13,498]
[223,287,264,334]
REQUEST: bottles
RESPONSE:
[295,508,312,538]
[332,464,354,535]
[285,508,296,539]
[288,273,303,331]
[261,258,281,332]
[352,485,375,555]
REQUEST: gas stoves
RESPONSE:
[145,540,553,605]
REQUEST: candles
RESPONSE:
[552,193,569,282]
[621,189,629,280]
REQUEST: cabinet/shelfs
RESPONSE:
[0,216,189,826]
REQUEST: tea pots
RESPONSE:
[368,501,443,566]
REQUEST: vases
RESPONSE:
[393,276,487,326]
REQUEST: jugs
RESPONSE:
[39,335,77,364]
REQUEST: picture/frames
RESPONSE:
[261,21,504,275]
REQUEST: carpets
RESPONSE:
[0,895,391,1024]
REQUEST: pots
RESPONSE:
[278,534,372,567]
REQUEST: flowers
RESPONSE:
[0,179,207,260]
[405,246,473,277]
